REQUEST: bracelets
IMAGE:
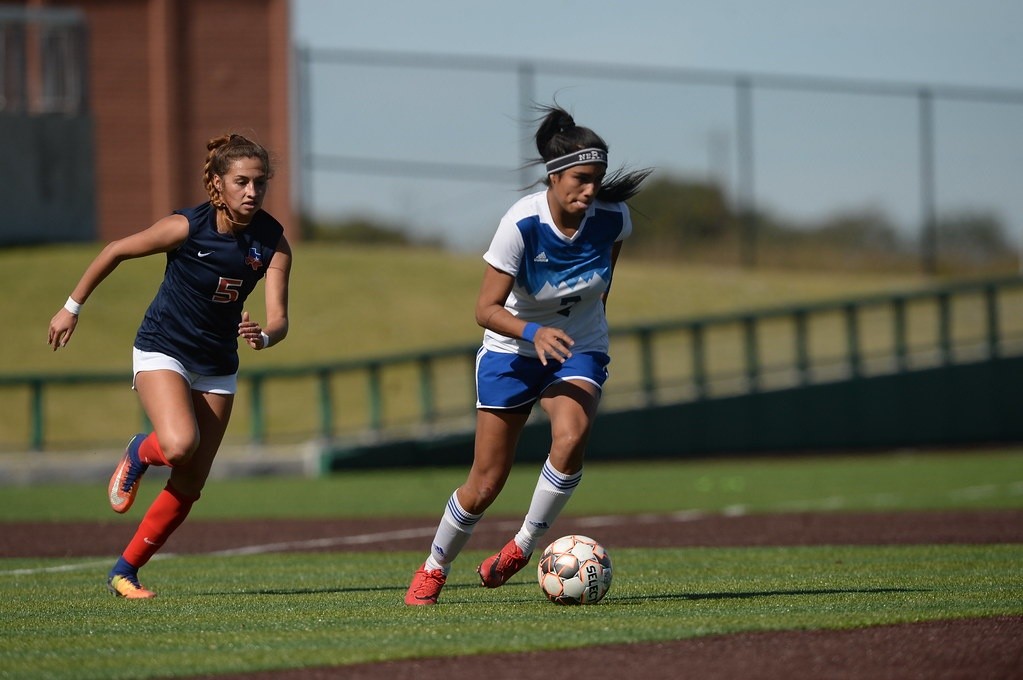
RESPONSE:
[259,328,269,347]
[63,296,83,318]
[522,321,540,342]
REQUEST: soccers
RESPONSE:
[537,534,613,606]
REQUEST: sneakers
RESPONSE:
[108,570,158,600]
[404,562,446,605]
[108,433,148,513]
[477,538,533,589]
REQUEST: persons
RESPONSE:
[50,128,292,601]
[403,90,653,606]
